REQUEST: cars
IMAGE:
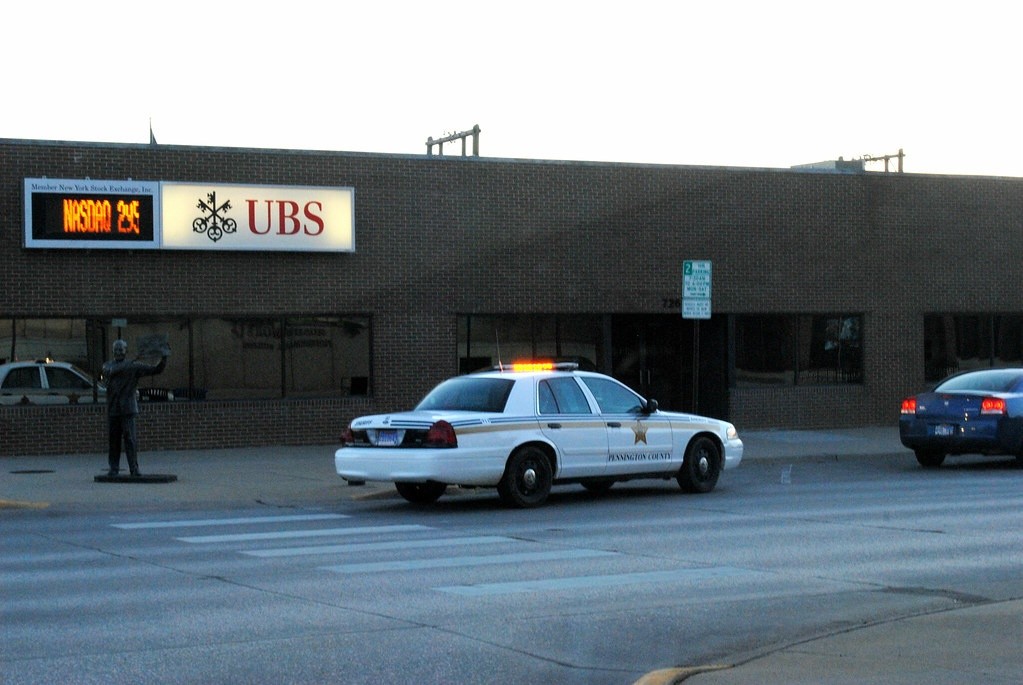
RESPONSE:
[334,362,743,508]
[899,368,1023,467]
[0,357,107,406]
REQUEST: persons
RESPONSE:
[102,340,169,478]
